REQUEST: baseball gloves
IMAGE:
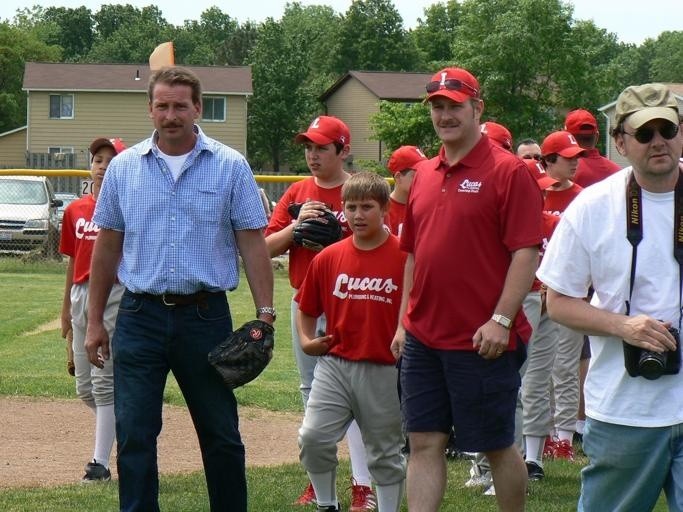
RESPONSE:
[288,203,344,253]
[208,320,275,389]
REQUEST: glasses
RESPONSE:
[425,78,478,94]
[626,124,676,144]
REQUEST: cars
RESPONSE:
[0,175,79,262]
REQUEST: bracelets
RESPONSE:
[255,306,276,321]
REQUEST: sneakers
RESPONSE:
[83,463,110,485]
[348,484,375,512]
[463,465,496,497]
[525,438,572,475]
[295,481,315,504]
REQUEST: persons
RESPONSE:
[58,136,128,485]
[263,67,683,512]
[84,67,276,511]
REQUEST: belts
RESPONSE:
[146,291,209,305]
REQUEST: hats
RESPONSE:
[387,145,425,174]
[566,109,598,134]
[519,159,559,190]
[479,121,511,149]
[609,83,682,133]
[421,68,481,104]
[295,116,350,149]
[541,132,587,161]
[90,138,125,154]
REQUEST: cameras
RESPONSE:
[622,327,682,381]
